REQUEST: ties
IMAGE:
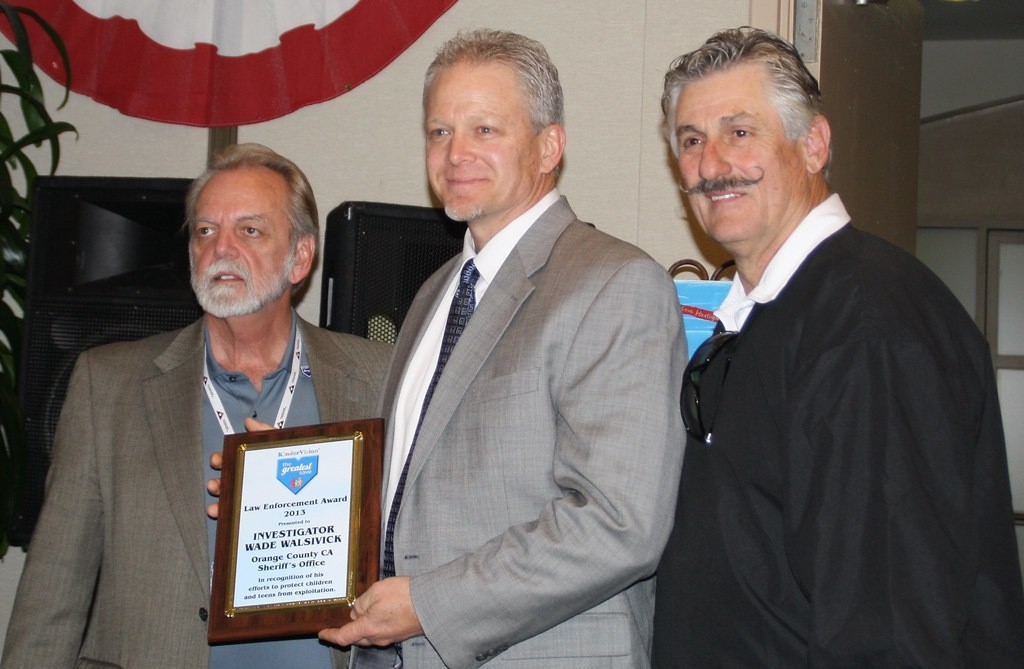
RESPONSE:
[381,258,481,667]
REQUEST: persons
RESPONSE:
[0,140,398,668]
[205,25,699,668]
[648,23,1024,669]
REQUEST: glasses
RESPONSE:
[680,331,746,446]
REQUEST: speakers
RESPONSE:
[320,201,469,347]
[8,176,206,548]
